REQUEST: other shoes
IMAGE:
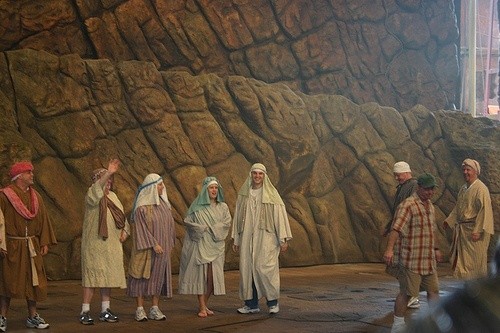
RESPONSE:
[390,315,406,333]
[407,297,420,308]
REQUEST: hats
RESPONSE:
[417,174,438,189]
[393,161,411,174]
[462,159,480,175]
[11,162,34,177]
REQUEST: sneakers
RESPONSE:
[98,308,119,322]
[26,313,49,329]
[269,303,279,313]
[79,311,94,325]
[135,310,148,322]
[0,315,8,332]
[149,307,166,321]
[237,306,260,314]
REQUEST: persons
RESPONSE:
[229,163,292,314]
[178,177,232,318]
[441,158,494,283]
[383,171,441,333]
[0,161,58,332]
[386,161,419,308]
[79,160,130,325]
[126,173,175,322]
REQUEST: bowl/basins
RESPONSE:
[488,105,499,115]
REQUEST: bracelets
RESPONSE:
[434,247,439,250]
